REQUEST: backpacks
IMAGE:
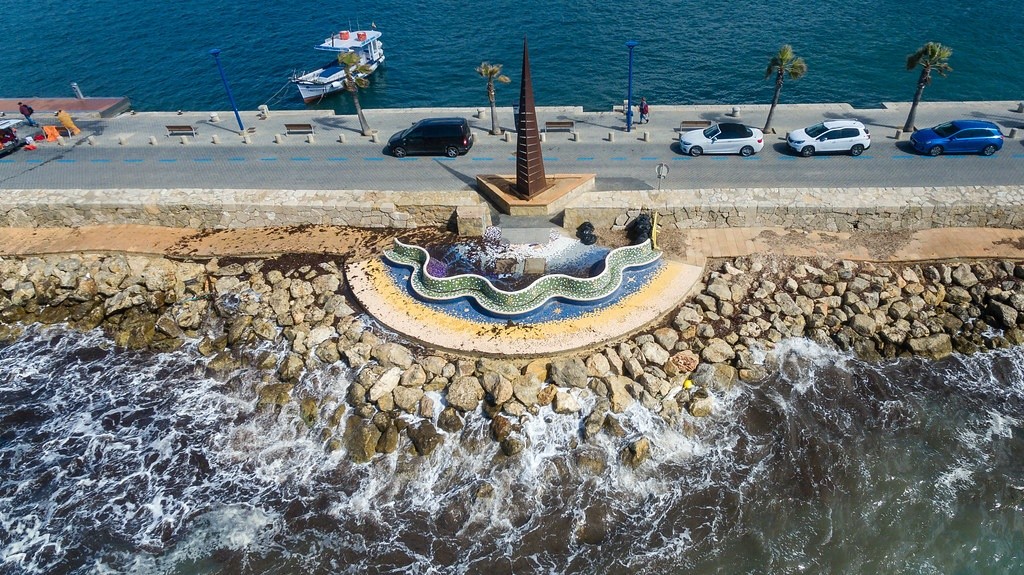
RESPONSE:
[23,105,34,114]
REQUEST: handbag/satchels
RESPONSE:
[640,102,648,114]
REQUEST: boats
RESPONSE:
[291,18,385,103]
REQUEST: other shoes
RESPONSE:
[638,122,642,124]
[29,124,33,126]
[646,119,649,123]
[36,124,39,128]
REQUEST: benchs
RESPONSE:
[283,123,316,137]
[680,120,711,131]
[545,121,575,133]
[166,125,199,137]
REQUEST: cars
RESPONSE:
[680,123,764,157]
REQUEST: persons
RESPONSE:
[18,101,39,127]
[639,97,650,124]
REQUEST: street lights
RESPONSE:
[624,41,640,132]
[209,49,247,136]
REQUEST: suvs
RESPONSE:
[787,118,871,157]
[910,120,1004,156]
[387,118,473,157]
